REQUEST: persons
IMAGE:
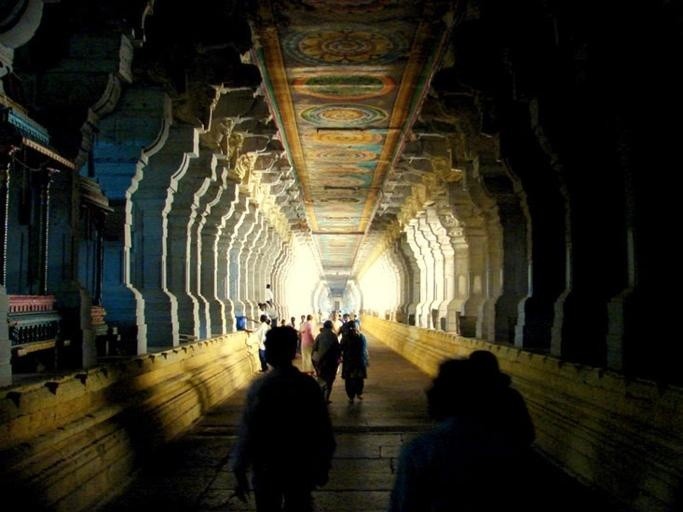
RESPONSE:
[387,358,542,512]
[236,300,361,374]
[338,320,368,402]
[466,350,535,449]
[265,284,273,307]
[310,320,340,404]
[230,325,339,512]
